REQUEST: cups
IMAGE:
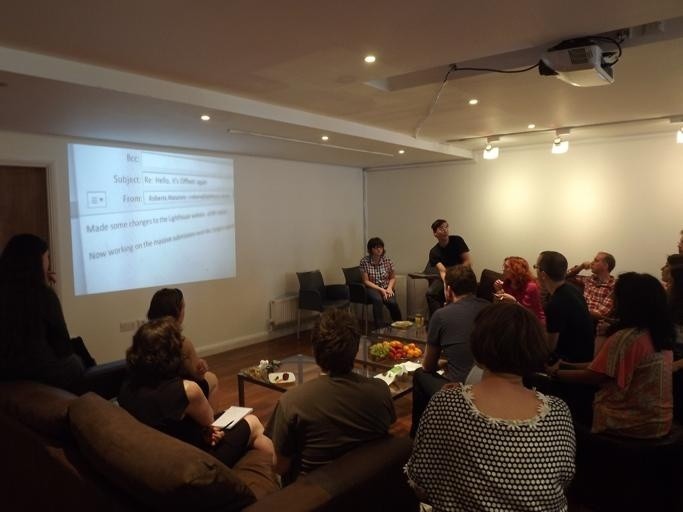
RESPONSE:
[415,314,426,328]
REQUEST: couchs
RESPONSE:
[1,378,419,512]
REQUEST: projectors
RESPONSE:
[538,45,615,89]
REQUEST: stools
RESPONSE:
[597,422,682,511]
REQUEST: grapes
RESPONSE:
[283,373,289,380]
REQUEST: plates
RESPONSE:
[395,361,426,372]
[269,372,296,383]
[391,320,413,329]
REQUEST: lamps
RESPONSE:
[482,128,572,160]
[670,117,683,143]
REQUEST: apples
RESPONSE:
[383,340,422,359]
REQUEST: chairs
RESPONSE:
[295,266,375,339]
[408,260,598,464]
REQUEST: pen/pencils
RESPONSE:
[220,420,234,432]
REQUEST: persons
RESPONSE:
[146,286,219,405]
[402,302,578,510]
[356,237,402,330]
[410,229,682,438]
[424,218,472,322]
[0,232,97,394]
[269,305,399,489]
[116,314,276,471]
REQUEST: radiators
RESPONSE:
[269,294,320,327]
